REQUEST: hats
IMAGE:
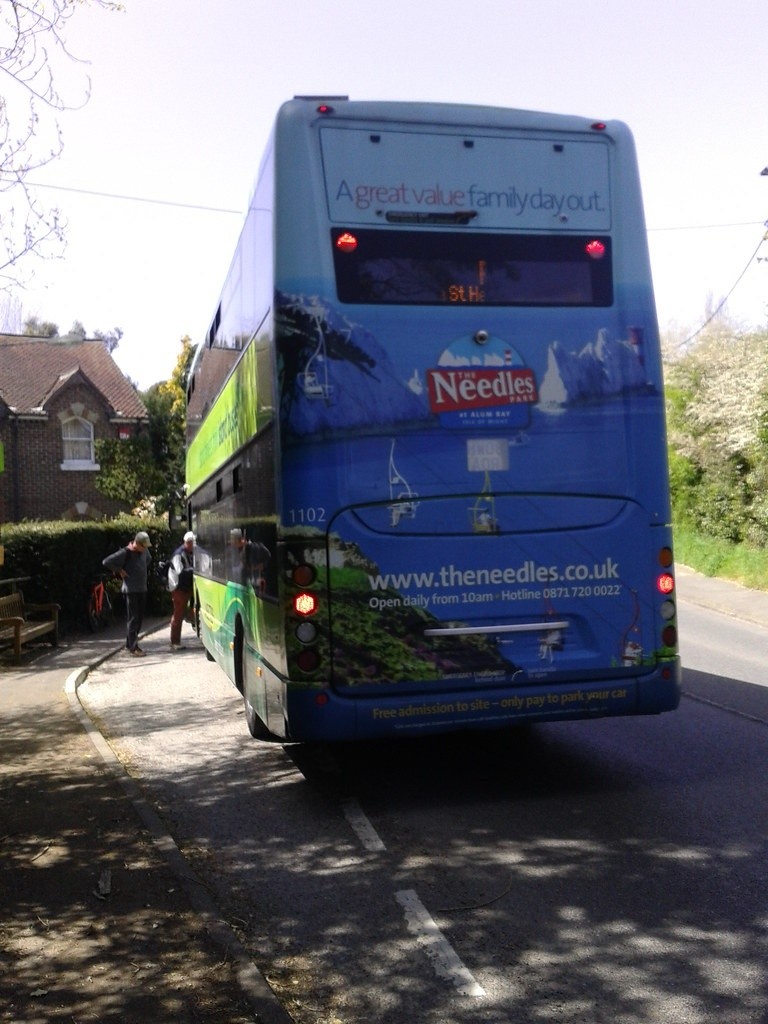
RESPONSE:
[135,531,153,547]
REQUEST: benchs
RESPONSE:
[0,589,62,664]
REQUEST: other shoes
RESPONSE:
[170,643,183,649]
[125,647,146,657]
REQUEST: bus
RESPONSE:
[166,88,678,738]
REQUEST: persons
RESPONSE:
[102,531,152,657]
[164,532,194,650]
